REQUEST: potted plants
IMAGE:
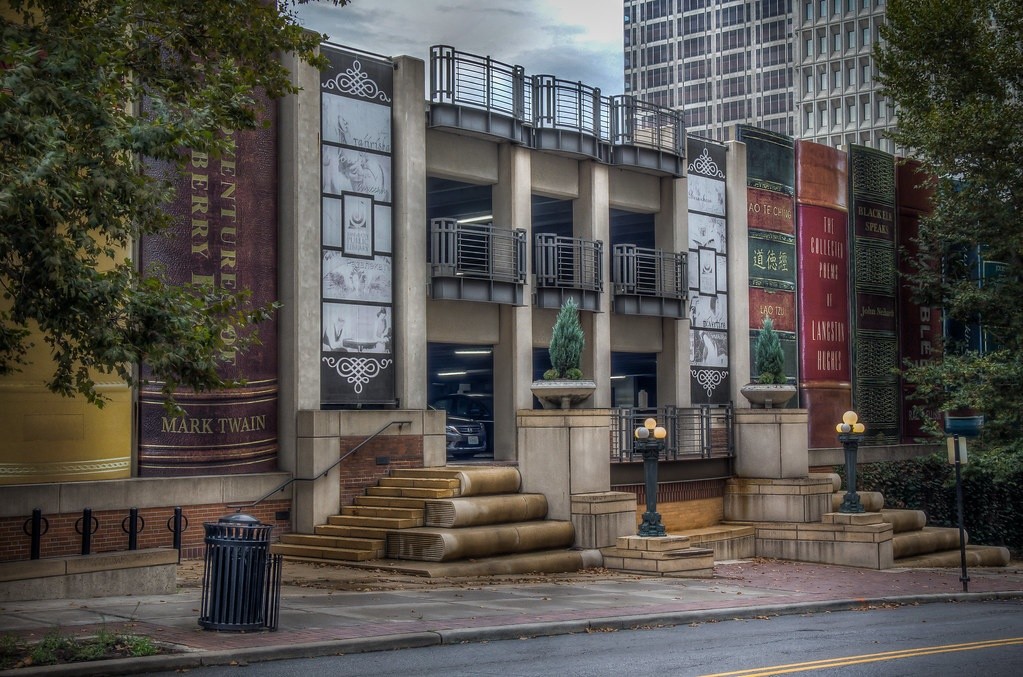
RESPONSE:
[740,315,797,408]
[529,294,596,409]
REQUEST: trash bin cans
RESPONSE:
[197,511,283,632]
